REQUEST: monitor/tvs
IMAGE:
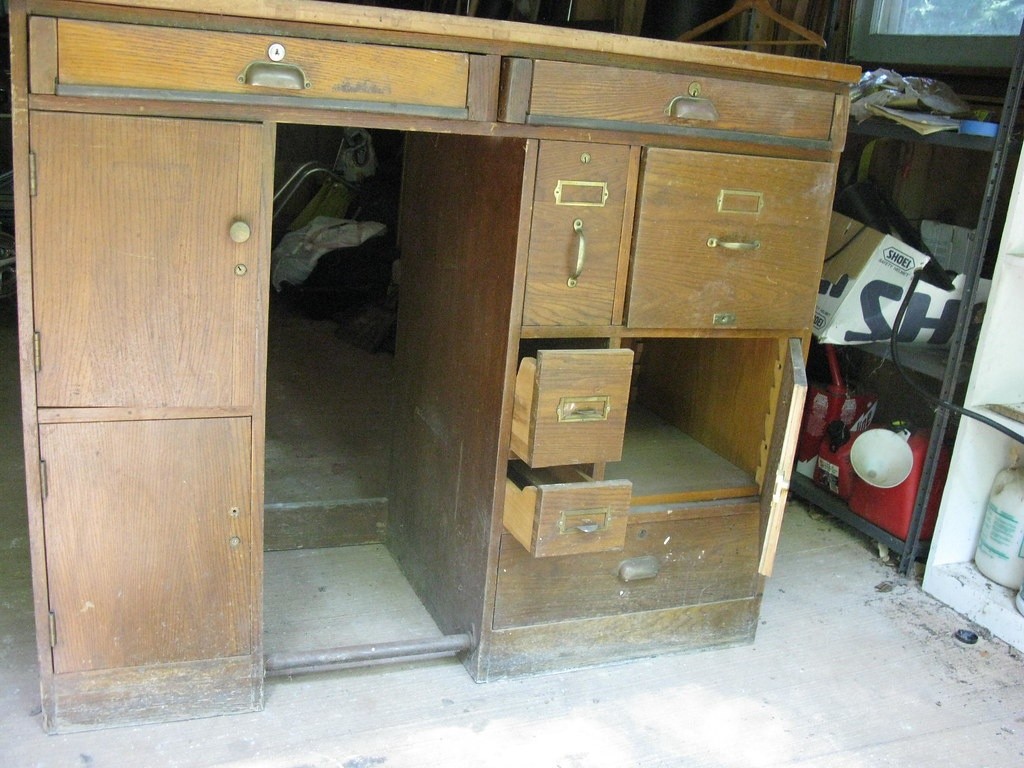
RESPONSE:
[849,0,1024,103]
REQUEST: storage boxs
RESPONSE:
[812,210,995,346]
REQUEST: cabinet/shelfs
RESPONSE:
[4,0,862,733]
[921,142,1024,654]
[787,19,1024,579]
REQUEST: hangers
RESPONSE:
[674,0,827,48]
